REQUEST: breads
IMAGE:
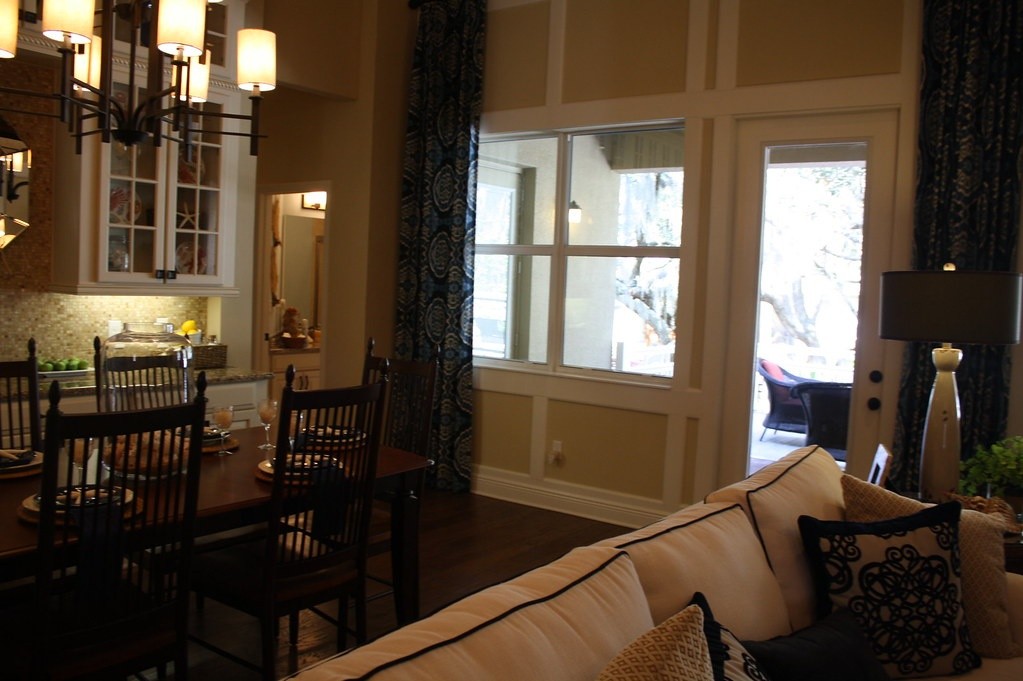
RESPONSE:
[104,429,190,476]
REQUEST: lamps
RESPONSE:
[0,0,278,156]
[0,146,32,204]
[878,264,1023,504]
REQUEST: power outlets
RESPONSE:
[552,440,563,453]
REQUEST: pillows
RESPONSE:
[841,474,1023,660]
[597,604,714,681]
[797,501,982,677]
[686,592,766,681]
[740,607,891,681]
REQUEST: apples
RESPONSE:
[37,358,90,371]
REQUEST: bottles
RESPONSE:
[208,335,217,345]
[108,235,129,271]
[202,332,207,345]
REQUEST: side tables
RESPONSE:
[902,492,1023,574]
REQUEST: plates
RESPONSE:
[22,485,134,513]
[108,185,141,224]
[258,453,343,476]
[178,149,205,185]
[0,452,43,469]
[37,367,95,378]
[102,460,188,480]
[176,431,230,447]
[175,242,206,275]
[301,425,366,443]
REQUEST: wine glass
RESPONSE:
[212,405,234,456]
[64,437,95,484]
[258,399,279,449]
[289,411,304,450]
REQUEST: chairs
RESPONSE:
[756,358,820,441]
[789,382,853,463]
[0,336,441,681]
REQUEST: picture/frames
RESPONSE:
[867,444,893,487]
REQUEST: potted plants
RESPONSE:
[955,436,1023,515]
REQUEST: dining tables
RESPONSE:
[0,425,434,628]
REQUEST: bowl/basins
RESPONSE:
[280,337,306,348]
[175,329,202,345]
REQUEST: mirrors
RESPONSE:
[0,117,31,250]
[281,215,324,332]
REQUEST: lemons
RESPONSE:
[176,320,198,334]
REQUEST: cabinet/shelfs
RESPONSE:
[133,381,272,432]
[0,393,133,451]
[44,35,242,298]
[270,353,321,425]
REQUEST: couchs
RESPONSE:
[280,444,1023,681]
